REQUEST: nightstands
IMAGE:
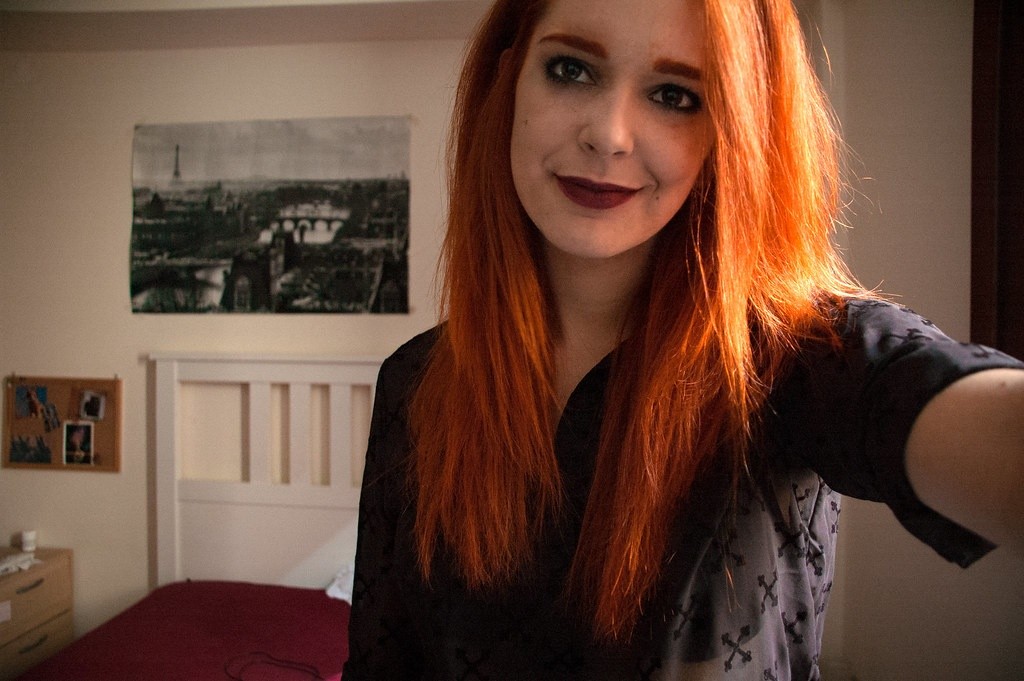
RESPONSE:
[0,546,73,681]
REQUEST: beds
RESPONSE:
[16,352,383,681]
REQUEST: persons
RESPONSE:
[339,0,1024,680]
